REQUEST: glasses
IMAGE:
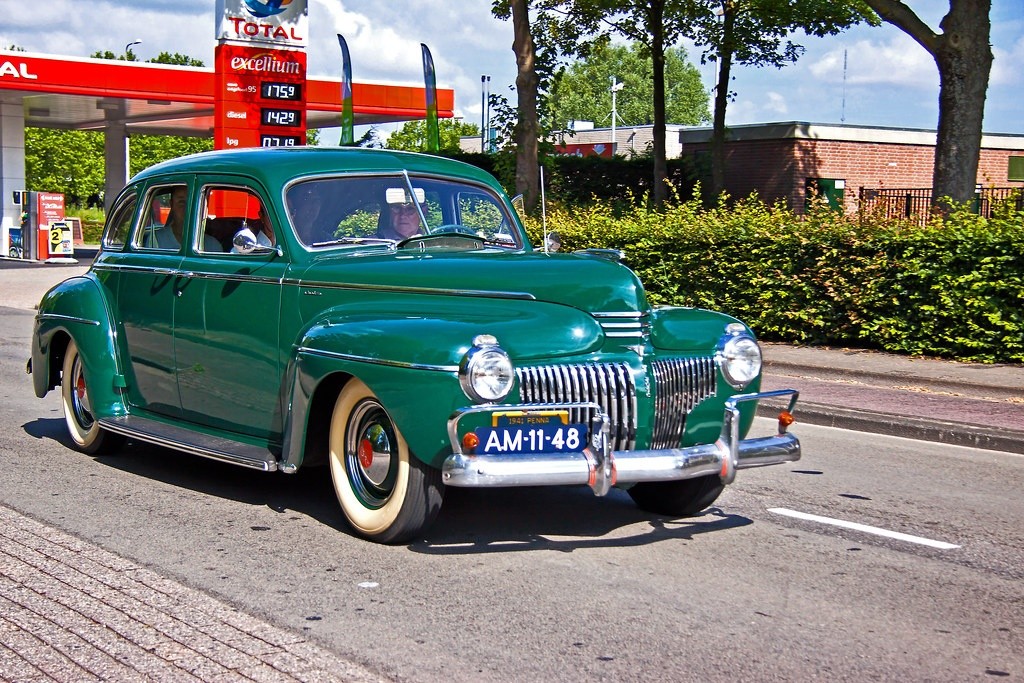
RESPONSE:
[263,208,297,218]
[392,203,418,215]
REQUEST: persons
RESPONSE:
[367,188,423,246]
[230,181,335,253]
[146,187,223,253]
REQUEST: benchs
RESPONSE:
[142,216,261,253]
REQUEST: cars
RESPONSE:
[26,146,802,544]
